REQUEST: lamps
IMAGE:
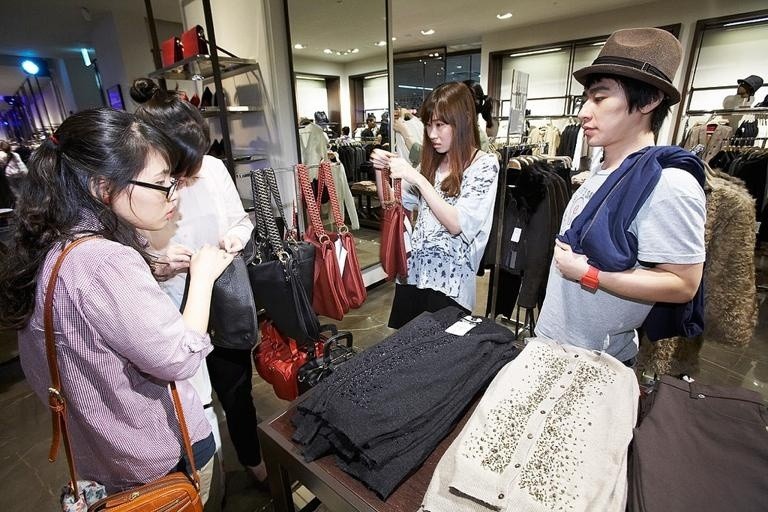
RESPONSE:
[18,57,53,80]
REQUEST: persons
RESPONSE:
[0,139,32,200]
[339,109,391,144]
[533,28,707,375]
[736,75,764,99]
[121,77,281,496]
[370,82,501,332]
[1,103,236,510]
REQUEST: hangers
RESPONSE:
[538,116,580,132]
[705,110,768,164]
[699,159,717,191]
[489,138,573,171]
[329,136,365,150]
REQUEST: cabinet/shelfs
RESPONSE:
[144,55,269,214]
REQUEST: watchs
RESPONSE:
[578,266,601,294]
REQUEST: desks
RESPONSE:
[255,340,527,512]
[347,185,383,232]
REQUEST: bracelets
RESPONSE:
[405,135,412,146]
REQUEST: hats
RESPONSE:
[737,75,763,96]
[573,28,682,105]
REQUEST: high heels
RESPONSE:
[180,87,224,108]
[207,138,224,158]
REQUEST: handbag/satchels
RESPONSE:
[314,111,329,123]
[87,472,204,512]
[208,256,258,351]
[247,162,367,400]
[375,157,412,279]
[162,25,208,68]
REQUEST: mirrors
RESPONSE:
[106,84,126,111]
[284,1,392,273]
